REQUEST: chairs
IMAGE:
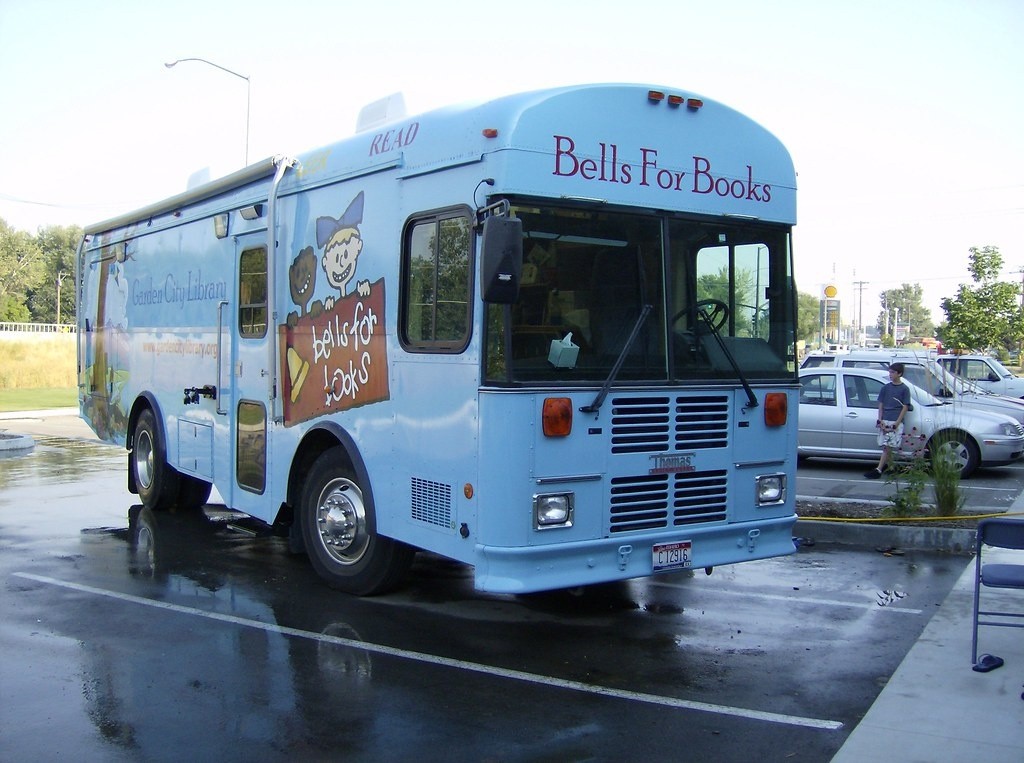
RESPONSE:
[846,387,861,405]
[971,518,1024,664]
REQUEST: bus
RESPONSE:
[76,79,805,599]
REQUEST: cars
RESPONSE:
[796,367,1024,477]
[799,351,1024,427]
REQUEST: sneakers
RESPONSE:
[864,468,881,478]
[881,464,889,473]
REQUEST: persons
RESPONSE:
[863,363,910,479]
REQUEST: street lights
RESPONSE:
[165,58,252,167]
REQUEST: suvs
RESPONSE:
[935,354,1024,400]
[829,344,844,351]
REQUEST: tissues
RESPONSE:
[548,331,580,370]
